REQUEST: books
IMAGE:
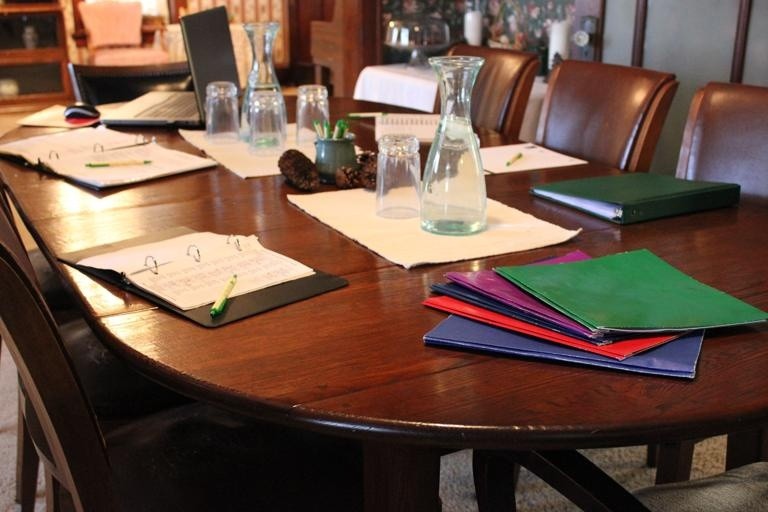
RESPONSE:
[53,223,350,331]
[528,170,745,226]
[423,315,705,377]
[422,296,690,360]
[444,249,604,338]
[430,282,626,344]
[474,140,590,178]
[492,248,768,329]
[373,110,482,148]
[0,125,221,193]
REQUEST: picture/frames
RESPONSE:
[67,44,194,100]
[0,75,767,512]
[352,61,549,141]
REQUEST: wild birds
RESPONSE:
[102,5,242,127]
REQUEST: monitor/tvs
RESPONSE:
[474,140,590,178]
[528,170,745,226]
[0,125,221,193]
[53,223,350,331]
[373,110,482,148]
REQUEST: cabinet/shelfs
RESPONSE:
[239,21,286,148]
[422,54,489,237]
[22,27,39,49]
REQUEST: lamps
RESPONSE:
[463,425,766,512]
[672,79,768,201]
[534,55,680,171]
[74,2,145,50]
[432,43,543,141]
[0,207,369,512]
[64,58,195,104]
[0,171,86,329]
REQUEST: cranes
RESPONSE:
[528,172,741,225]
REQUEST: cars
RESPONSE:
[295,84,330,145]
[313,132,357,184]
[204,81,241,145]
[375,136,424,219]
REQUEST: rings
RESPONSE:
[209,274,237,318]
[346,111,389,118]
[86,160,152,168]
[312,118,350,143]
[506,153,522,166]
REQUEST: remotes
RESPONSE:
[64,101,100,118]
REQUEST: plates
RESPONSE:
[0,0,78,122]
